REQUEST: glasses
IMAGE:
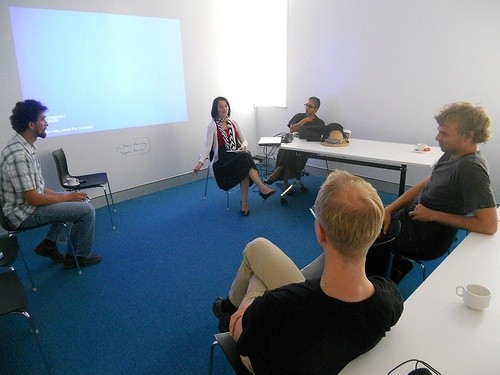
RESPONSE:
[310,205,323,231]
[304,104,315,109]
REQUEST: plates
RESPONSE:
[64,183,80,186]
[413,148,432,153]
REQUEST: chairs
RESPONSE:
[209,216,426,375]
[52,148,116,230]
[0,216,82,375]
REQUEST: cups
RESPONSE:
[417,143,427,151]
[456,284,492,311]
[344,130,351,139]
[67,178,79,185]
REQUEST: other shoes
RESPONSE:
[264,176,278,185]
[390,257,413,284]
[212,296,230,333]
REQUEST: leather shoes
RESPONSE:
[35,243,64,263]
[64,254,102,268]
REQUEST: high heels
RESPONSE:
[240,200,249,216]
[259,190,276,200]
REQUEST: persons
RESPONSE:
[0,100,102,269]
[211,169,404,375]
[301,101,498,281]
[264,97,325,186]
[194,97,277,216]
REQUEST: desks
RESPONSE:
[258,136,280,178]
[338,206,500,375]
[279,138,445,199]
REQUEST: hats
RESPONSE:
[321,130,350,147]
